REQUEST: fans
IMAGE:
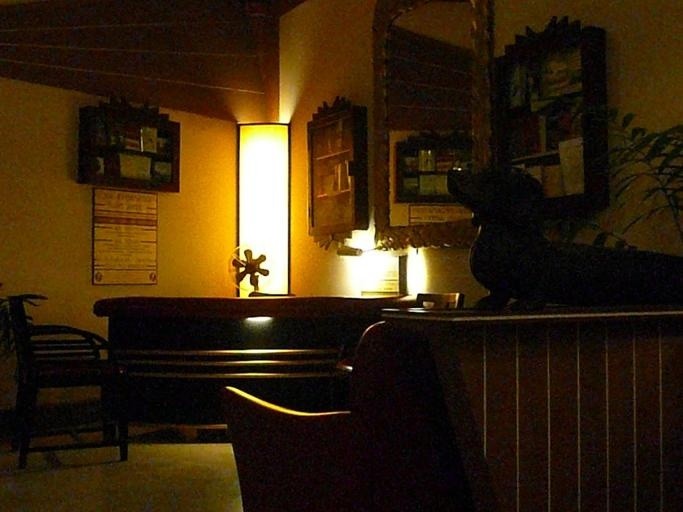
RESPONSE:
[228,246,276,296]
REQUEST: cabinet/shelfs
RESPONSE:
[307,94,369,249]
[81,95,179,192]
[499,15,609,216]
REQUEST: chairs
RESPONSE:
[10,295,129,465]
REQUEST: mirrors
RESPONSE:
[368,0,495,248]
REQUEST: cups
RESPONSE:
[423,301,435,310]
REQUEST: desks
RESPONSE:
[93,297,421,423]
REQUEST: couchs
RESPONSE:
[227,313,683,512]
